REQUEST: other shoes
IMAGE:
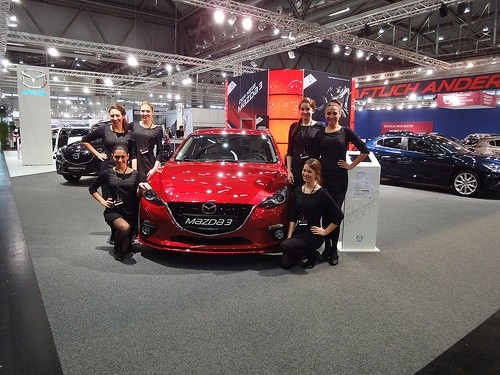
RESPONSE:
[114,245,123,261]
[319,246,332,261]
[329,246,339,266]
[109,234,116,245]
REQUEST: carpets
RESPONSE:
[9,171,500,375]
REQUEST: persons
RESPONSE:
[80,105,130,245]
[175,125,185,137]
[312,100,369,266]
[88,144,141,262]
[286,96,326,192]
[279,158,344,270]
[164,125,173,138]
[130,100,163,181]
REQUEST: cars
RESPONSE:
[364,132,500,197]
[54,137,108,183]
[429,133,500,153]
[135,126,293,256]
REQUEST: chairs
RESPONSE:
[200,140,222,159]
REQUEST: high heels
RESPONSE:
[303,249,321,269]
